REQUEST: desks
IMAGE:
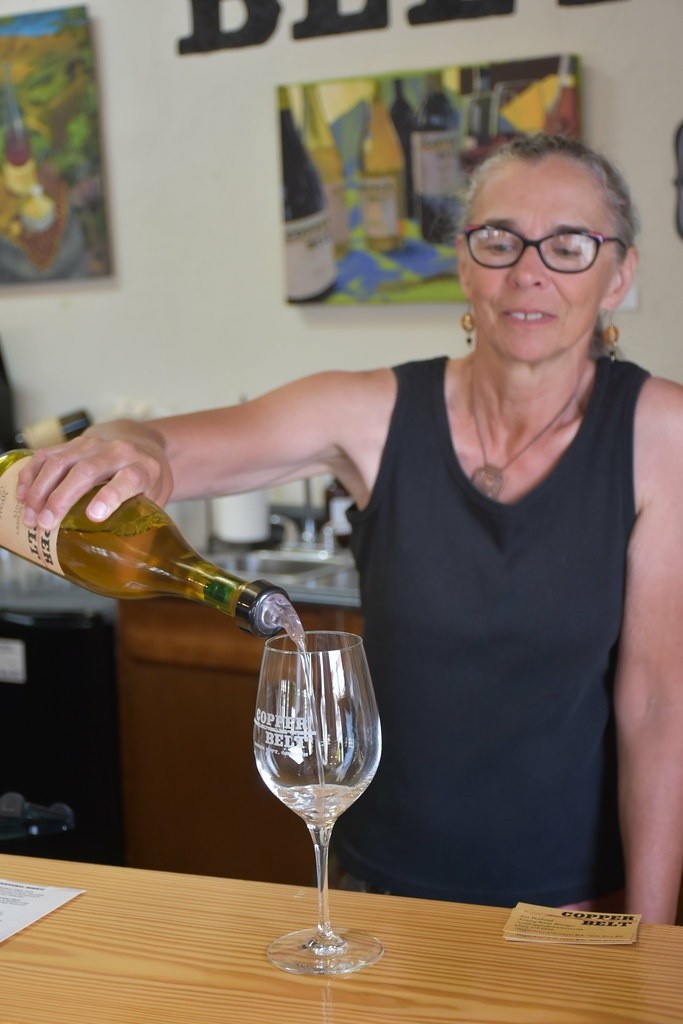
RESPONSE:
[0,855,683,1023]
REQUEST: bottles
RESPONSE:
[355,83,406,255]
[383,76,473,245]
[296,85,350,256]
[0,448,293,638]
[0,409,94,456]
[277,86,338,303]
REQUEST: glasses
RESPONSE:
[462,224,627,273]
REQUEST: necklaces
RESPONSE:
[469,352,588,501]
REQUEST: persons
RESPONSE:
[16,133,683,925]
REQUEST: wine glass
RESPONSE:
[253,631,386,975]
